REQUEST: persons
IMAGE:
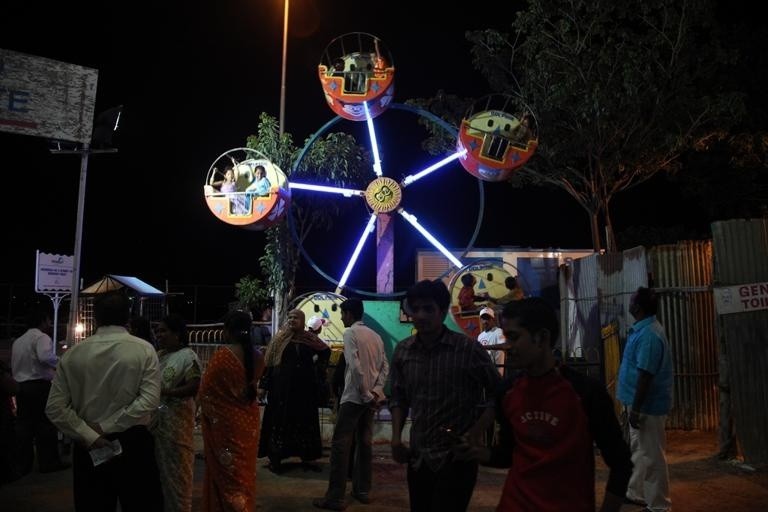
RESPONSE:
[43,291,165,511]
[1,361,20,485]
[459,274,486,315]
[258,309,331,474]
[211,157,245,214]
[486,115,535,156]
[245,166,272,216]
[496,298,634,512]
[156,315,202,511]
[313,296,390,510]
[486,277,526,304]
[200,311,265,511]
[614,285,676,511]
[477,306,505,379]
[329,35,392,92]
[11,307,67,473]
[388,278,502,512]
[131,316,155,345]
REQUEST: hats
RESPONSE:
[480,307,495,319]
